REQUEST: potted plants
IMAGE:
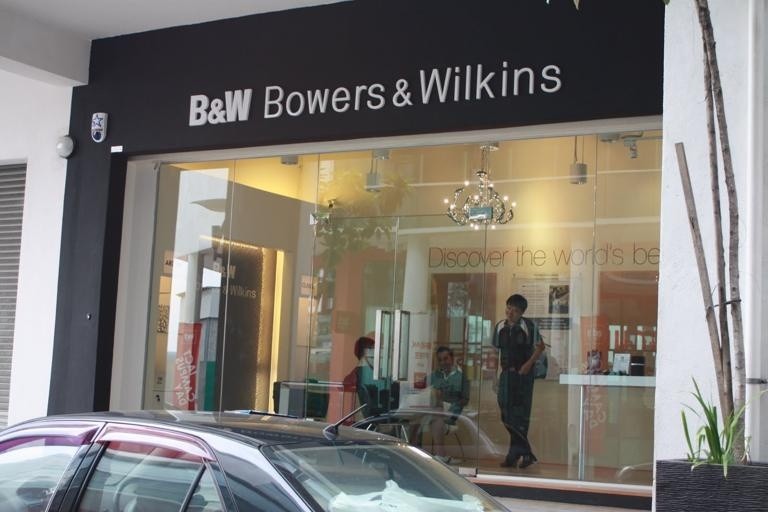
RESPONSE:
[655,372,768,512]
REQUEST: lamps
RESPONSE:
[569,135,587,186]
[443,142,514,228]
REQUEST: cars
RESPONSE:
[0,403,515,512]
[349,400,521,467]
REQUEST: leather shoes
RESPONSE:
[519,453,537,468]
[501,461,517,467]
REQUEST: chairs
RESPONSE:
[347,375,469,466]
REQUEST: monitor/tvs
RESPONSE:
[611,353,632,374]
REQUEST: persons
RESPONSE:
[343,337,405,435]
[490,294,544,470]
[409,346,469,464]
